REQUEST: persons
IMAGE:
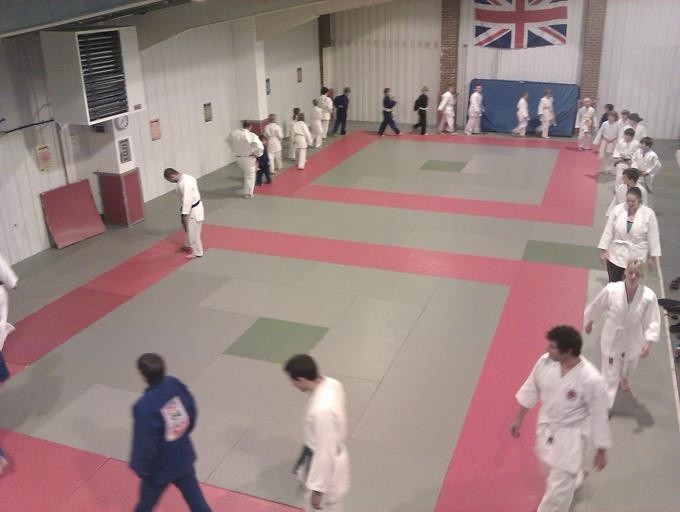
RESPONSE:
[591,104,663,218]
[596,187,663,288]
[281,353,352,511]
[307,100,325,150]
[0,252,19,354]
[126,353,211,510]
[377,87,404,137]
[574,97,597,152]
[464,84,487,136]
[535,89,558,139]
[509,326,614,512]
[510,92,531,137]
[291,112,314,170]
[225,121,265,197]
[163,168,208,260]
[265,113,285,173]
[319,87,334,141]
[584,258,660,419]
[334,86,351,134]
[254,133,272,187]
[287,108,304,163]
[437,84,462,135]
[412,85,431,136]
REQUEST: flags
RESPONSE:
[473,0,569,50]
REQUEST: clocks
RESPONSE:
[115,114,130,130]
